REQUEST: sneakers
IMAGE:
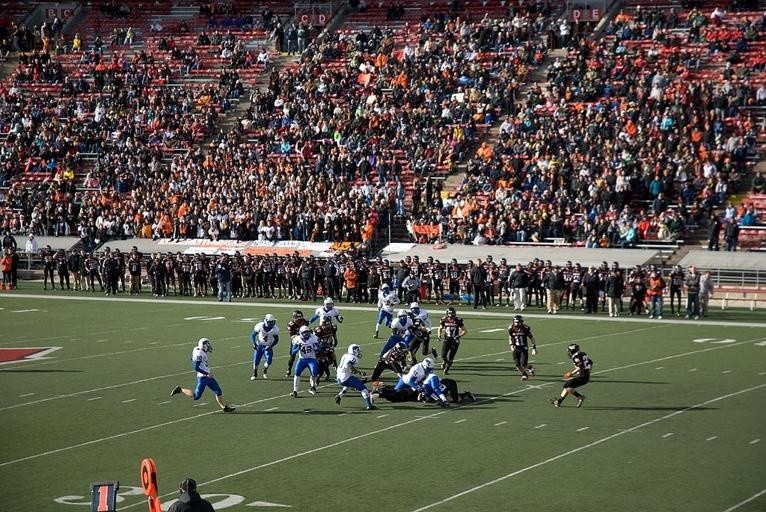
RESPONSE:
[577,395,585,408]
[440,360,447,369]
[529,364,535,377]
[520,375,528,381]
[170,385,181,397]
[223,405,235,412]
[445,367,449,374]
[251,368,379,411]
[466,391,478,401]
[551,399,560,407]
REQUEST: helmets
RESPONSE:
[381,282,436,370]
[567,344,579,358]
[445,307,456,319]
[513,315,523,329]
[198,297,363,357]
[179,478,196,493]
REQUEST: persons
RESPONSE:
[1,1,766,320]
[285,297,344,398]
[167,478,215,512]
[334,344,383,412]
[171,338,236,414]
[551,344,593,408]
[373,283,476,408]
[250,313,280,382]
[507,315,537,381]
